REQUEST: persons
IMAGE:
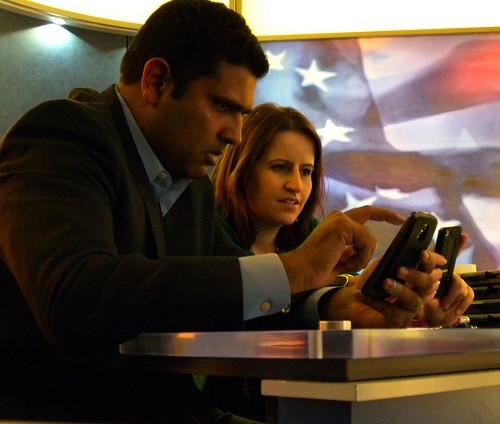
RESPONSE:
[0,2,438,424]
[200,102,476,424]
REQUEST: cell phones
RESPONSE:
[361,211,439,300]
[433,225,462,298]
